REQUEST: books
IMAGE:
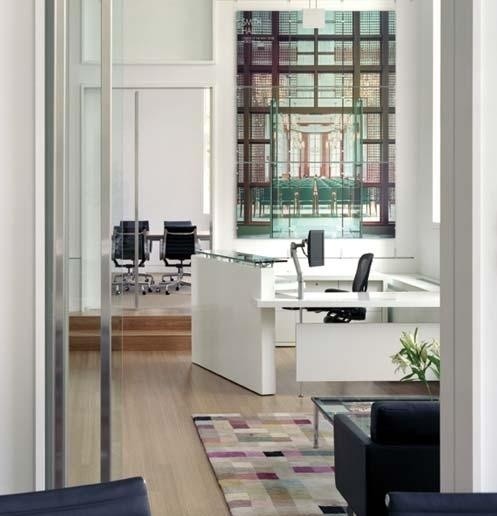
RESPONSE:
[342,401,375,417]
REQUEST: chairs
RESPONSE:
[114,218,211,295]
[332,394,440,516]
[321,251,374,324]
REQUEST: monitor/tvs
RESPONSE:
[308,230,325,267]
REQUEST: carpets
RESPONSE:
[192,398,356,515]
